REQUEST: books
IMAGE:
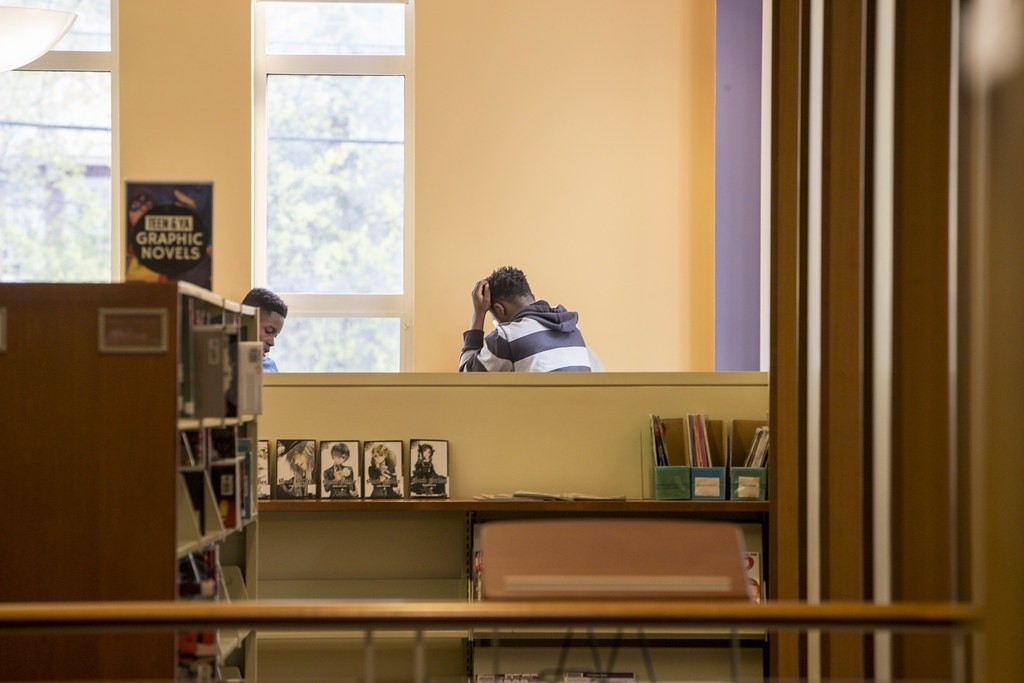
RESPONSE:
[211,435,256,528]
[745,424,768,468]
[236,344,264,417]
[652,414,671,466]
[689,414,713,468]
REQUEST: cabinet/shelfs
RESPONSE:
[0,282,260,682]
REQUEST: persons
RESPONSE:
[458,265,592,373]
[241,288,288,372]
